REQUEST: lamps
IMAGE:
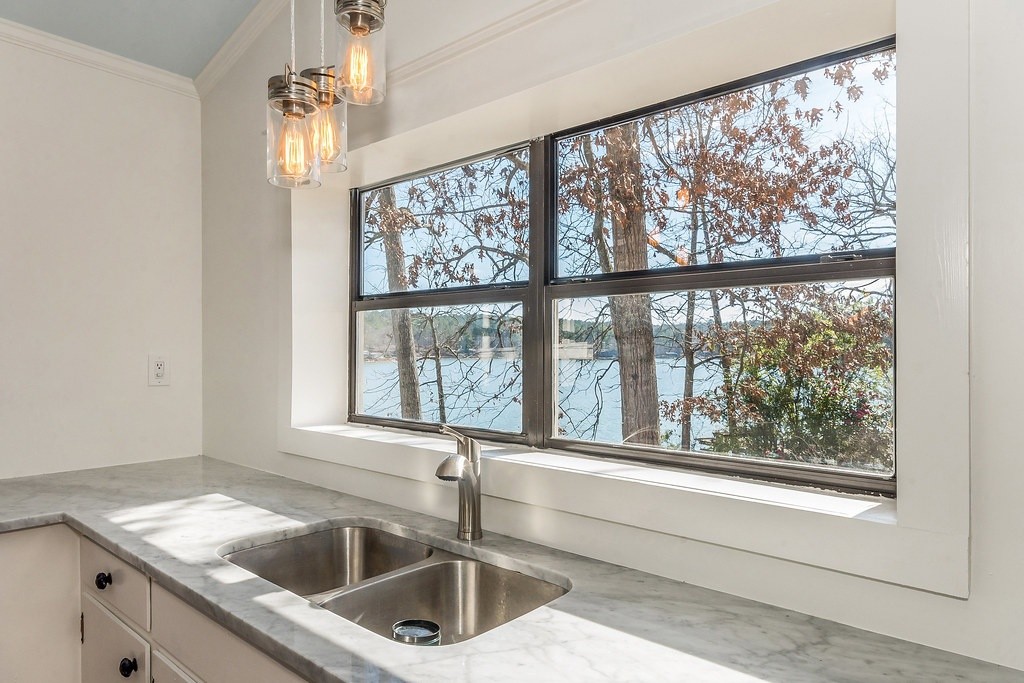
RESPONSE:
[332,0,387,106]
[265,0,322,191]
[299,0,349,174]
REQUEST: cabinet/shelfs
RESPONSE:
[78,535,309,683]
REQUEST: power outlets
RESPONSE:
[147,352,170,386]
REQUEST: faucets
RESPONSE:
[435,425,483,541]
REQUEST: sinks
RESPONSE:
[215,516,436,598]
[318,560,574,648]
